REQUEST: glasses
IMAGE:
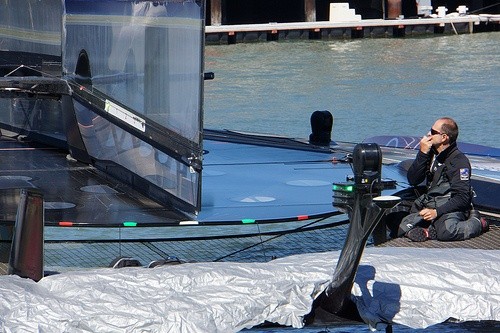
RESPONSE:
[431,128,442,135]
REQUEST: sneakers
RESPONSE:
[407,227,437,242]
[480,214,489,233]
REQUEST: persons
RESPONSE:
[385,117,489,242]
